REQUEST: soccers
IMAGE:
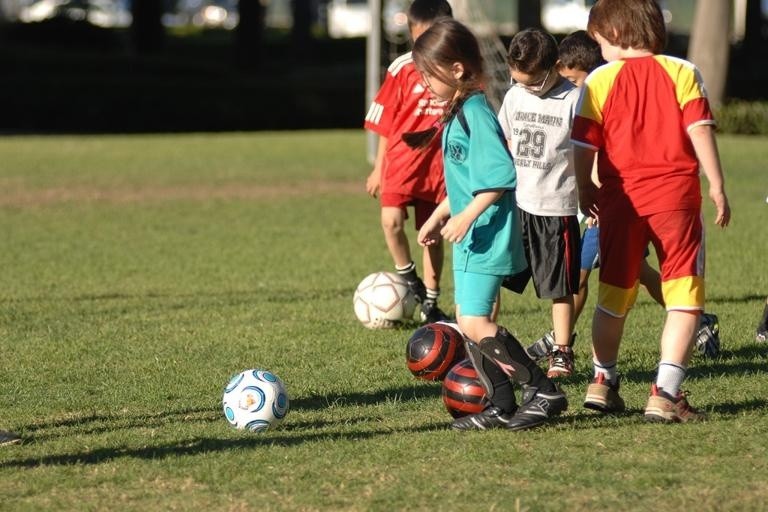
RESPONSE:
[406,324,466,381]
[443,358,493,418]
[222,369,289,433]
[352,271,414,329]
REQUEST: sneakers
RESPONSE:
[505,386,569,431]
[406,278,426,305]
[523,327,578,363]
[583,372,626,415]
[641,383,707,426]
[545,349,576,379]
[416,298,439,325]
[696,312,721,359]
[451,406,512,434]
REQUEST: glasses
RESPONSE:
[509,71,551,94]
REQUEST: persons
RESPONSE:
[401,22,570,433]
[489,30,601,382]
[363,0,484,324]
[522,29,727,369]
[571,4,733,425]
